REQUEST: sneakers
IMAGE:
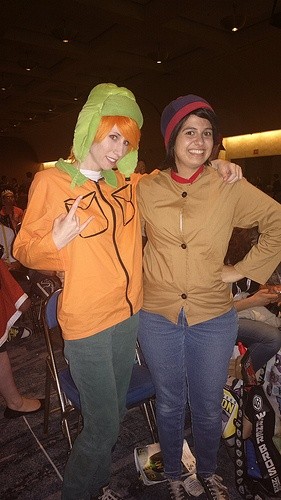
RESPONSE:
[92,481,129,500]
[4,325,33,343]
[196,473,231,500]
[166,478,191,500]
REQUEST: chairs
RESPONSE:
[42,287,156,452]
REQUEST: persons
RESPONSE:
[1,82,281,500]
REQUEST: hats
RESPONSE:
[1,189,14,200]
[160,94,216,155]
[69,82,144,191]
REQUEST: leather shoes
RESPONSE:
[3,398,46,420]
